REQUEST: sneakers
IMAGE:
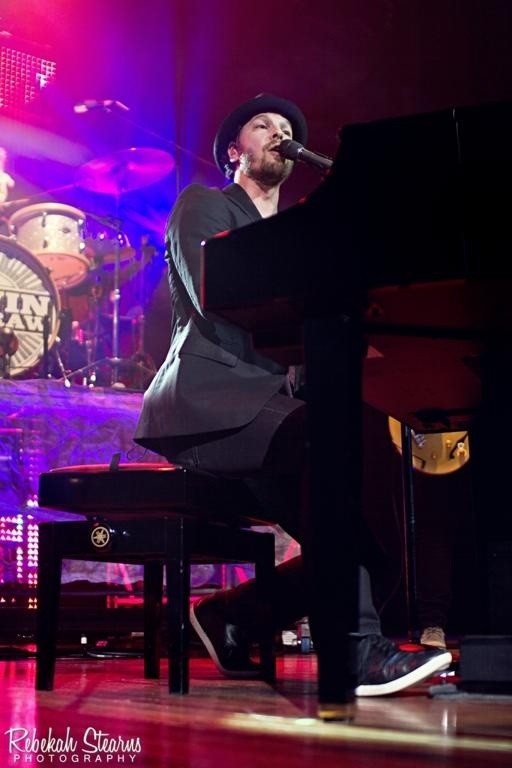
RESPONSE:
[189,595,264,676]
[346,633,453,697]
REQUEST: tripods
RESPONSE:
[59,237,161,390]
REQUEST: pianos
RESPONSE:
[202,105,510,699]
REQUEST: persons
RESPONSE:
[128,91,454,700]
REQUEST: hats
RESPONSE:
[213,94,308,179]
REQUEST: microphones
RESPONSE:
[279,139,333,171]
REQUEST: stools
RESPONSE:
[36,460,276,694]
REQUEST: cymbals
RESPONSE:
[86,238,135,265]
[76,147,174,194]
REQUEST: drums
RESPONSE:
[10,202,91,290]
[0,234,63,377]
